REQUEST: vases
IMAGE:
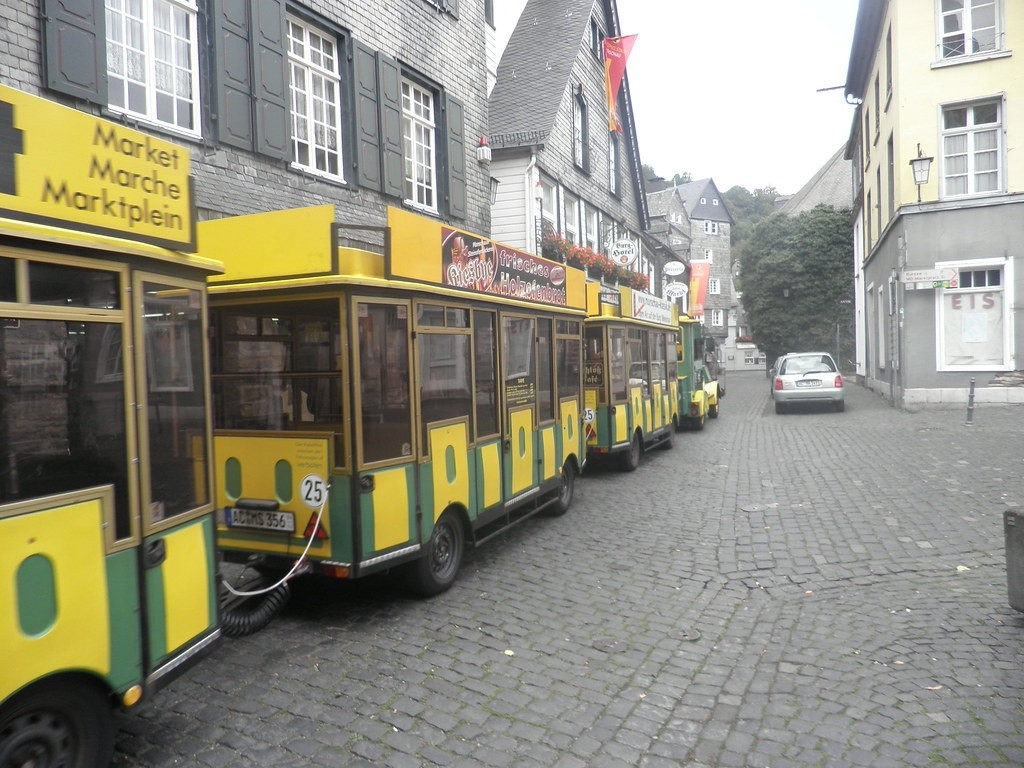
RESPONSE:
[587,267,602,280]
[617,278,628,287]
[542,249,563,263]
[604,274,615,286]
[566,258,585,271]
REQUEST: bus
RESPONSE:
[0,84,721,768]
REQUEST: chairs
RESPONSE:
[6,450,82,500]
[83,460,181,538]
[787,362,800,371]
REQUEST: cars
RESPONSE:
[769,352,845,415]
[768,355,783,396]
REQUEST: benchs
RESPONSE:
[377,397,550,444]
[293,421,409,466]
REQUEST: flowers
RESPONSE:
[540,229,651,292]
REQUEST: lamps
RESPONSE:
[909,142,935,203]
[848,359,861,367]
